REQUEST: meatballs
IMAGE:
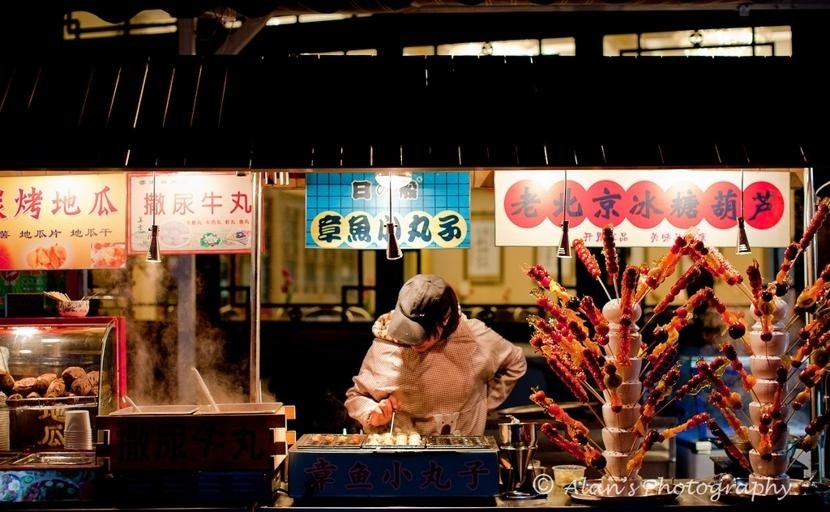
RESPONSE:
[312,432,421,445]
[158,222,189,246]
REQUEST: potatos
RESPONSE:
[0,366,108,399]
[26,243,66,270]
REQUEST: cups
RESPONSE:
[63,408,94,452]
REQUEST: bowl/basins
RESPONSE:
[57,296,92,318]
[551,463,590,488]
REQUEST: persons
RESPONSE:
[343,273,528,438]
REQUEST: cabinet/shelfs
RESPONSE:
[0,314,128,449]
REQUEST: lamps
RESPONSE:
[144,172,162,262]
[735,169,751,255]
[556,171,572,259]
[384,170,403,260]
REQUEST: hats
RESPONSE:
[387,273,454,346]
